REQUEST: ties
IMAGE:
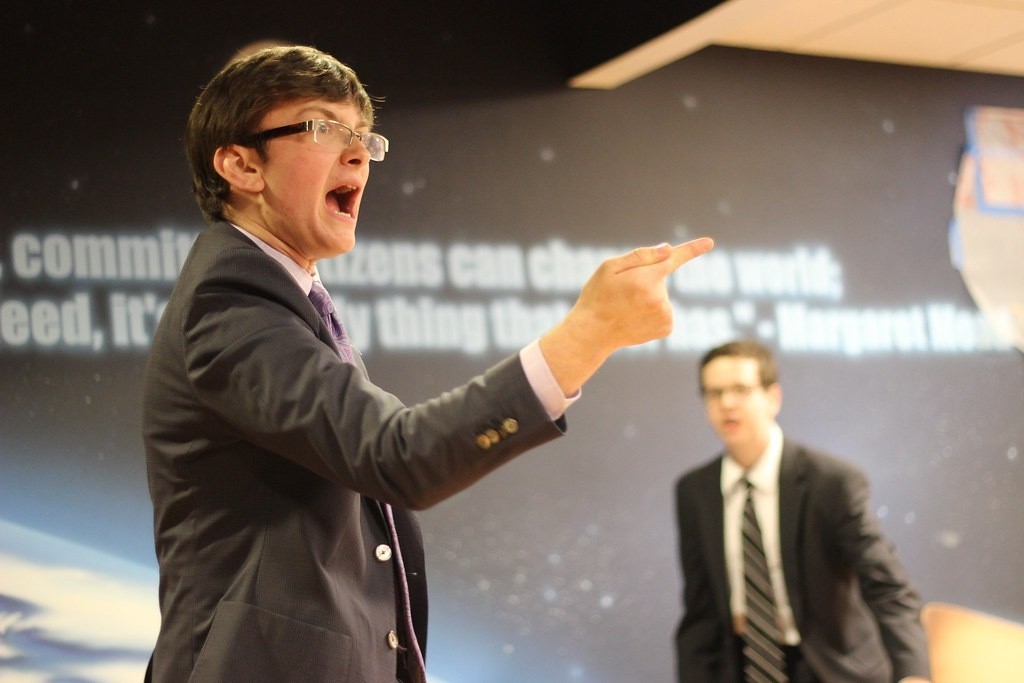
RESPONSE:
[308,277,428,683]
[740,478,790,683]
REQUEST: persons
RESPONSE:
[673,340,934,683]
[142,47,714,683]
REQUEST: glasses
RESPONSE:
[703,380,768,401]
[237,115,392,162]
